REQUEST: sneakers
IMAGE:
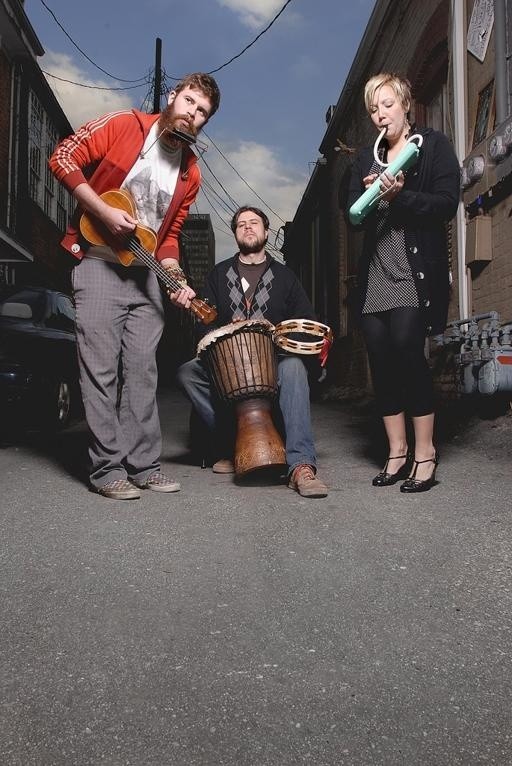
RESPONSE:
[98,479,141,500]
[141,472,181,493]
[212,457,234,474]
[287,463,328,497]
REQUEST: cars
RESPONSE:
[0,282,84,431]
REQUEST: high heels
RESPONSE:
[400,444,439,493]
[372,445,412,487]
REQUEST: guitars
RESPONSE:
[81,190,218,326]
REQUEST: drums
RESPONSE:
[195,319,286,482]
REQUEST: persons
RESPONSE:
[345,72,460,492]
[47,73,221,500]
[177,206,329,498]
